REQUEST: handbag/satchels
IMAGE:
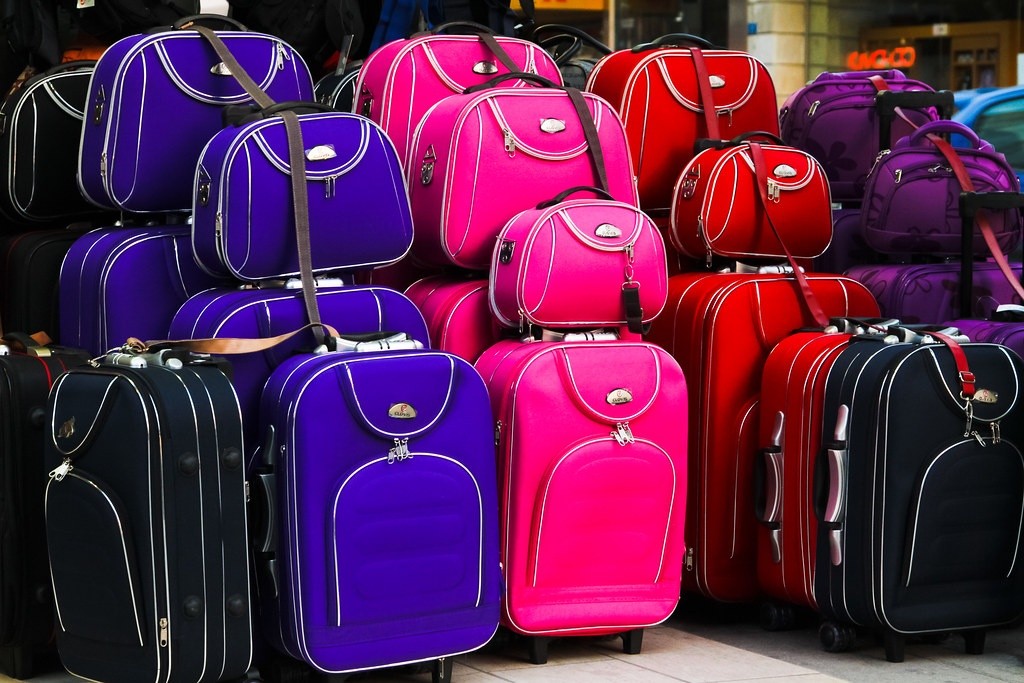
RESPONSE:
[402,72,639,272]
[314,59,365,112]
[193,101,415,282]
[350,20,565,179]
[859,121,1024,256]
[583,33,779,209]
[488,185,668,339]
[76,14,318,214]
[668,131,833,269]
[0,59,121,229]
[779,69,941,200]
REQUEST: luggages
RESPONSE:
[0,90,1024,683]
